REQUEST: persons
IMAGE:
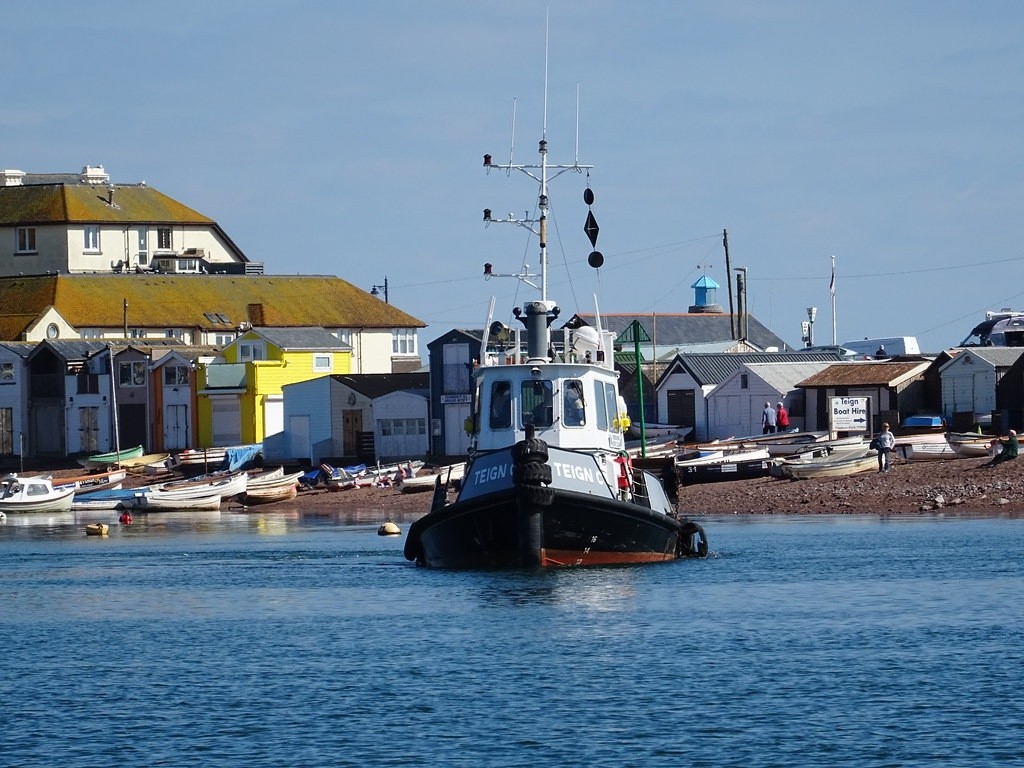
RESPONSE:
[762,402,777,435]
[989,429,1018,467]
[875,345,887,360]
[877,423,895,473]
[393,464,414,487]
[776,403,789,432]
[532,402,545,424]
[564,382,584,425]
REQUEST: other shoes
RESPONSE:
[885,469,888,473]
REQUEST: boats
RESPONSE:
[399,461,467,492]
[178,444,265,473]
[629,421,694,443]
[0,468,127,496]
[20,453,425,507]
[404,2,707,575]
[86,522,109,536]
[0,472,76,514]
[74,444,144,471]
[944,430,1024,457]
[135,490,222,512]
[619,433,957,481]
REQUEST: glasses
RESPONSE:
[884,425,889,428]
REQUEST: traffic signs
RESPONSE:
[829,396,870,432]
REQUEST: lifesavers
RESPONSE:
[403,521,419,562]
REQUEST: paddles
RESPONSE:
[376,459,381,480]
